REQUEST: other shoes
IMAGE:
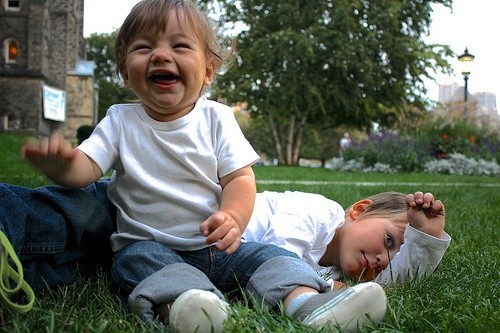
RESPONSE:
[168,290,234,333]
[293,281,387,333]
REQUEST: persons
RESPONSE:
[0,180,451,310]
[21,0,387,333]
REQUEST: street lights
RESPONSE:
[457,45,475,102]
